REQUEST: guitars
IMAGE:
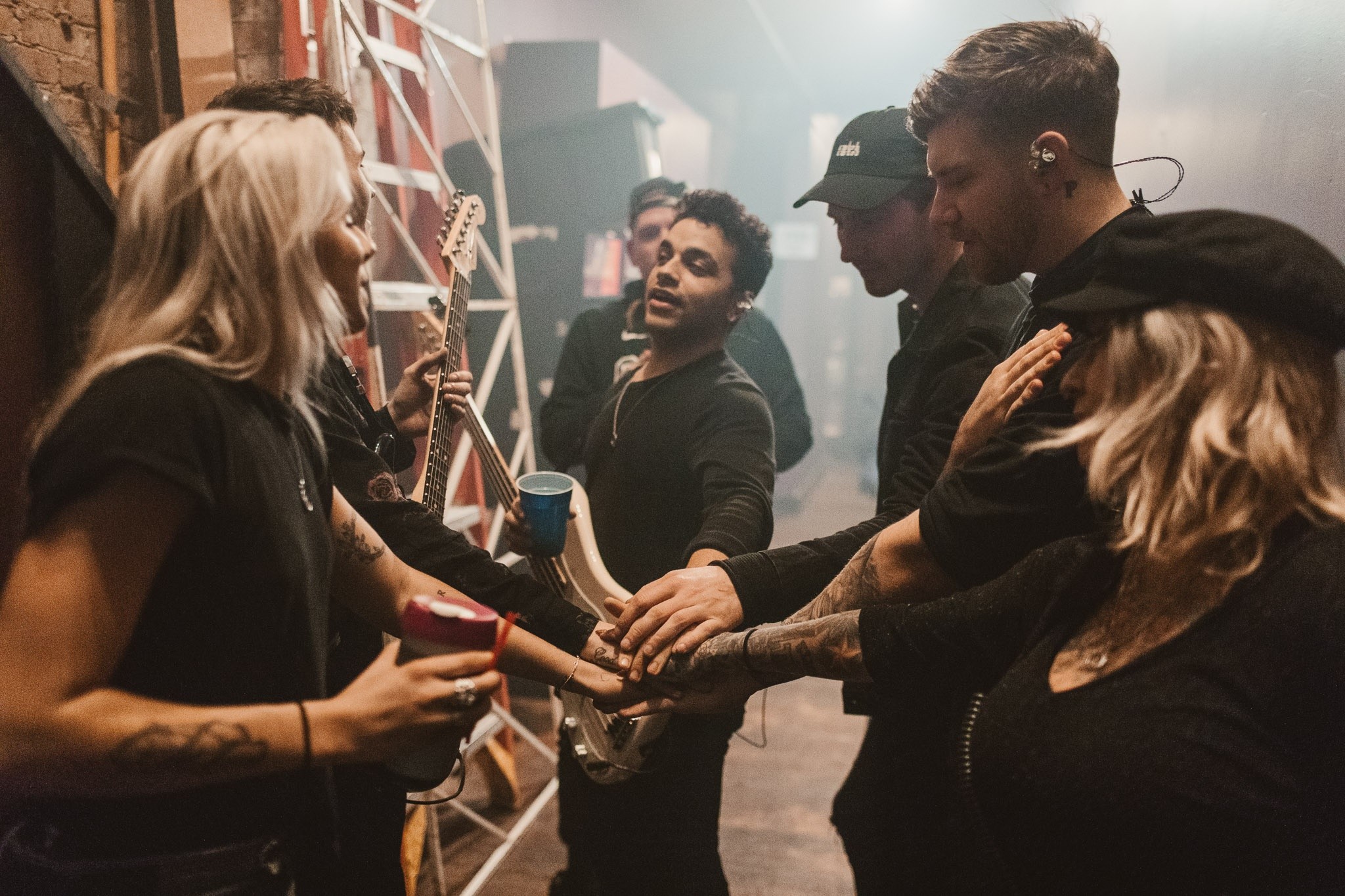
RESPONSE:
[414,292,677,787]
[332,187,488,795]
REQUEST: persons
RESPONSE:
[538,177,813,896]
[1,78,722,896]
[595,22,1345,896]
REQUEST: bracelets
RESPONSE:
[743,628,758,671]
[561,655,580,688]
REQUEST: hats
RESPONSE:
[793,103,930,210]
[629,176,690,228]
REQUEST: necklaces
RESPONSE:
[610,364,677,447]
[1083,556,1176,670]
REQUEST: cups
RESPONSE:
[515,471,573,555]
[377,596,498,784]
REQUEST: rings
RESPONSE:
[454,677,476,706]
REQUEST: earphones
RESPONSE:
[1028,147,1055,177]
[735,300,752,311]
